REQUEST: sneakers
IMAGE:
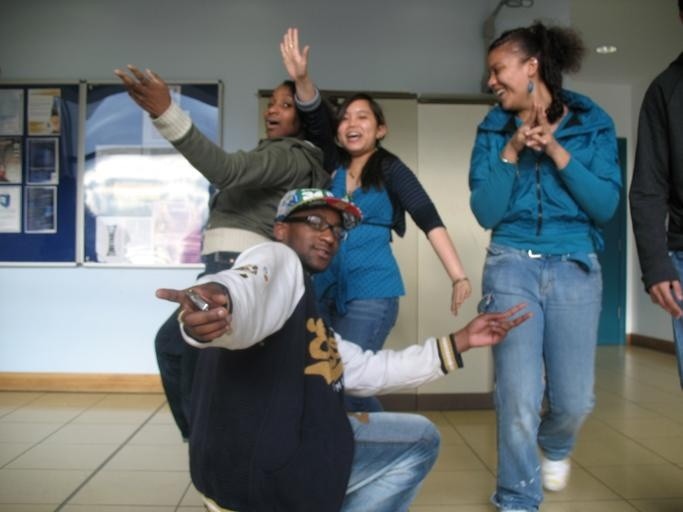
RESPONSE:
[540,454,572,493]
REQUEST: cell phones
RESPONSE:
[183,288,209,312]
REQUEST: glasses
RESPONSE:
[281,213,350,242]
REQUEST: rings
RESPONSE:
[288,44,293,48]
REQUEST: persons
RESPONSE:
[279,27,474,413]
[467,20,624,512]
[154,187,538,511]
[629,0,682,391]
[112,64,331,445]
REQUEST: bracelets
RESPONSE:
[451,277,469,286]
[500,153,512,164]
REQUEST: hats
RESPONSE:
[272,187,364,224]
